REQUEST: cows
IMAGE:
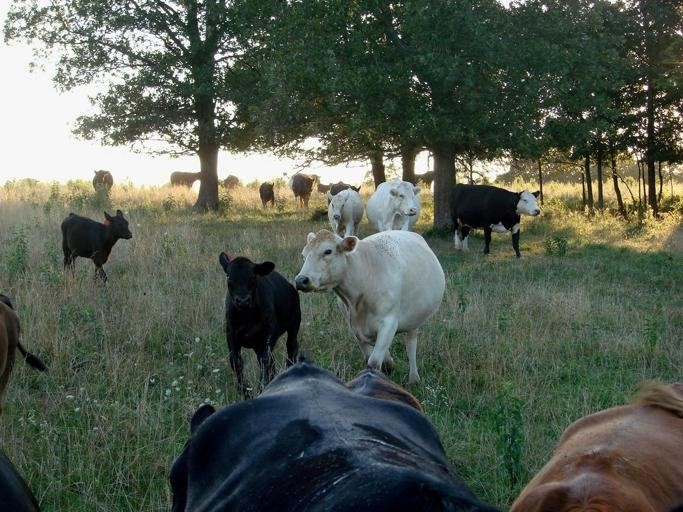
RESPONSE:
[61,209,132,288]
[326,188,364,239]
[224,175,239,189]
[219,252,301,401]
[0,293,49,416]
[0,449,42,512]
[414,171,434,190]
[92,169,113,193]
[508,379,683,512]
[328,181,362,207]
[294,228,446,392]
[292,174,315,209]
[449,183,540,260]
[343,368,424,412]
[170,170,202,190]
[317,183,333,194]
[365,179,421,233]
[259,182,274,211]
[169,361,501,512]
